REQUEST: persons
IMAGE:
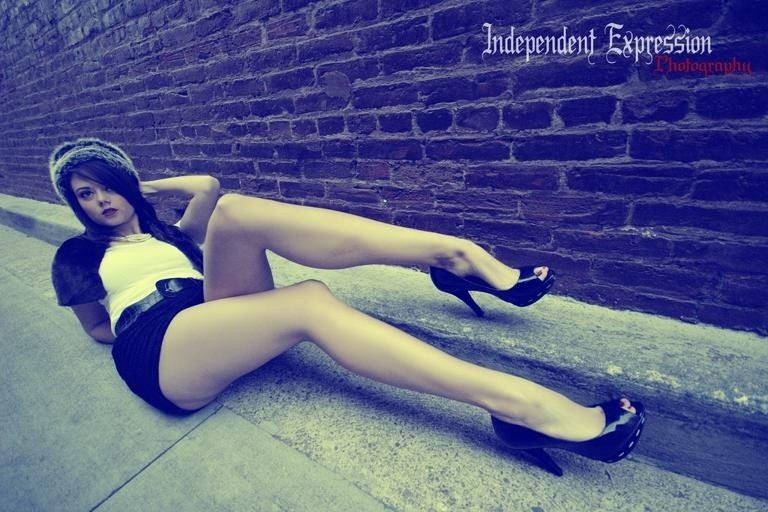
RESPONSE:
[49,136,645,476]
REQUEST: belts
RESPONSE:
[114,277,199,336]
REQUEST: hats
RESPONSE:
[48,137,139,204]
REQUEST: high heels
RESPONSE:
[490,398,645,476]
[431,262,556,317]
